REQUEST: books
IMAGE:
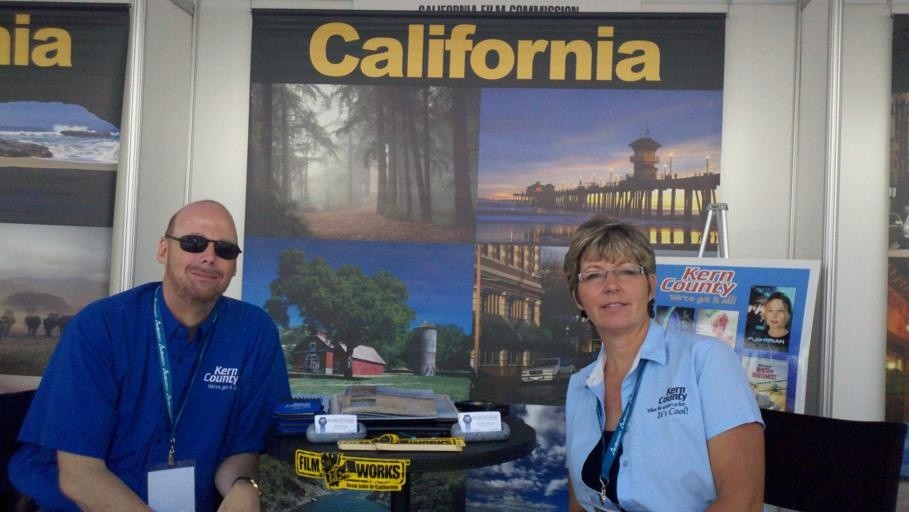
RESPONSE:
[276,396,325,437]
[329,384,461,440]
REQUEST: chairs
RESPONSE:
[0,390,43,512]
[760,408,909,512]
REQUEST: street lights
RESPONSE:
[579,168,617,186]
[663,164,667,175]
[668,152,673,177]
[705,156,710,176]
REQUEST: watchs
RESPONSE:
[231,477,262,497]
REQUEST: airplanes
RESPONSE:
[757,378,787,395]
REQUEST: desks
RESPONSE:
[267,414,538,512]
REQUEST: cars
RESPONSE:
[520,357,576,382]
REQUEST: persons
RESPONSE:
[563,212,767,512]
[8,198,294,512]
[763,291,792,347]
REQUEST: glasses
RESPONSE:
[577,265,647,282]
[165,233,240,260]
